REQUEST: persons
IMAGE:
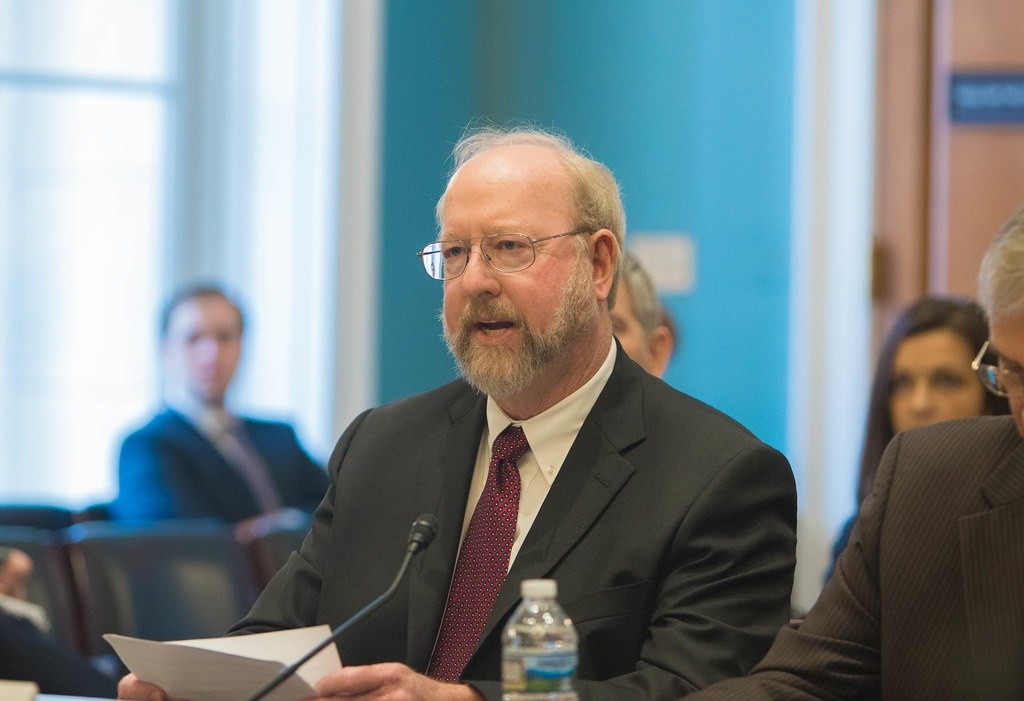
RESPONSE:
[119,284,330,575]
[817,293,1009,583]
[610,245,674,378]
[116,117,799,701]
[0,548,118,699]
[681,203,1024,701]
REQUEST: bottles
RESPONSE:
[500,578,583,701]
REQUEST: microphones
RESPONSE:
[247,514,441,701]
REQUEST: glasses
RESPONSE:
[973,341,1024,398]
[413,228,591,281]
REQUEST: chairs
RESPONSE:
[0,507,313,658]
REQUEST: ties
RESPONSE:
[425,425,532,685]
